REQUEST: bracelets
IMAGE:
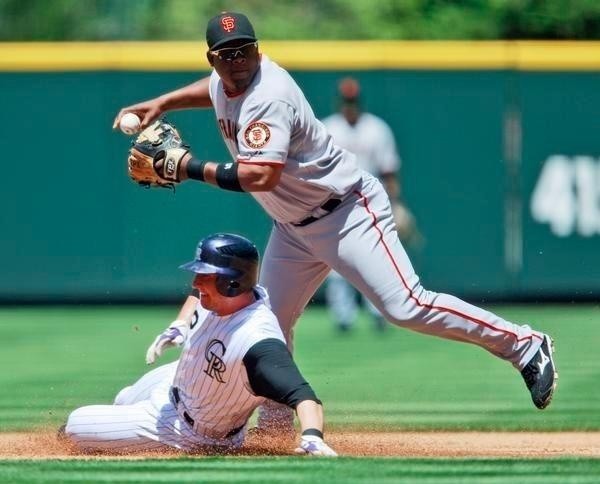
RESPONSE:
[302,428,323,439]
[185,156,206,182]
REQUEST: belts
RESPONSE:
[172,387,246,438]
[289,199,342,226]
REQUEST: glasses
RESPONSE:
[208,42,258,61]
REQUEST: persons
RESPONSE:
[109,10,559,442]
[55,231,338,459]
[314,74,401,334]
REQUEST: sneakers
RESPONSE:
[521,334,558,410]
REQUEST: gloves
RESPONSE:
[295,427,337,457]
[146,320,188,365]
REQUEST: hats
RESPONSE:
[206,10,256,50]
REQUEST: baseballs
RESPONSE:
[119,113,140,135]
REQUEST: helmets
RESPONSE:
[177,232,259,296]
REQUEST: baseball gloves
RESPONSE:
[130,120,191,190]
[392,201,415,239]
